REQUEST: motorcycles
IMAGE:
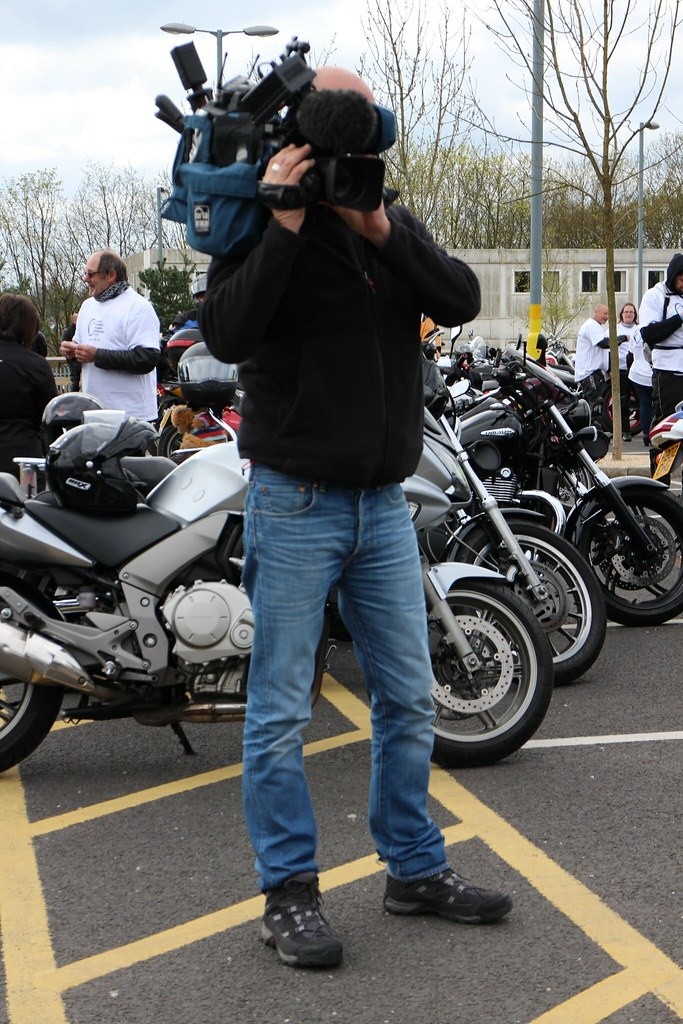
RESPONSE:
[0,332,683,766]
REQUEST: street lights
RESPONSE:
[156,186,166,268]
[157,20,279,100]
[635,121,661,316]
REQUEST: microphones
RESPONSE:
[297,87,379,156]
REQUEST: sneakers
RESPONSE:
[384,868,514,925]
[260,872,344,968]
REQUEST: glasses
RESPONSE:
[84,268,107,276]
[624,311,635,313]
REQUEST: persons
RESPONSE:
[61,314,82,392]
[59,250,160,422]
[575,304,630,404]
[639,253,683,487]
[606,303,638,442]
[421,317,442,359]
[169,273,208,330]
[627,324,653,446]
[198,66,513,967]
[0,294,64,483]
[30,333,47,358]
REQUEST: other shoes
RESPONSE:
[642,433,652,447]
[623,431,632,441]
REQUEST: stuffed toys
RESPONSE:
[171,404,227,449]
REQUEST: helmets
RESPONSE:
[40,393,104,457]
[45,409,179,521]
[178,342,239,409]
[190,273,208,311]
[166,328,205,374]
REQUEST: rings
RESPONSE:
[272,163,280,170]
